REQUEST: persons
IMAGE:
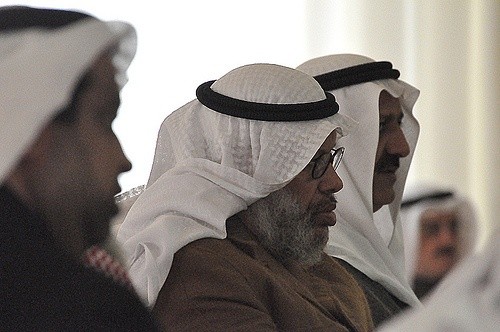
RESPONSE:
[0,6,160,332]
[110,54,500,332]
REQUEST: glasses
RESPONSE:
[311,147,346,179]
